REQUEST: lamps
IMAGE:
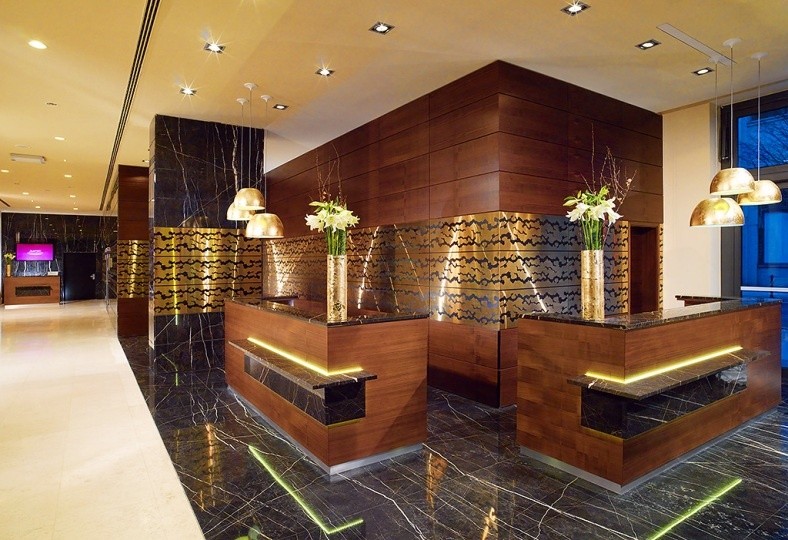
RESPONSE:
[688,54,746,228]
[736,51,784,206]
[710,36,756,195]
[246,94,285,239]
[234,82,267,212]
[226,97,256,221]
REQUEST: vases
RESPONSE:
[580,249,606,321]
[6,264,11,278]
[326,254,349,322]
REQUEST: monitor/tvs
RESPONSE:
[16,242,55,262]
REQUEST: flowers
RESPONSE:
[5,254,16,265]
[560,125,638,251]
[304,196,360,254]
